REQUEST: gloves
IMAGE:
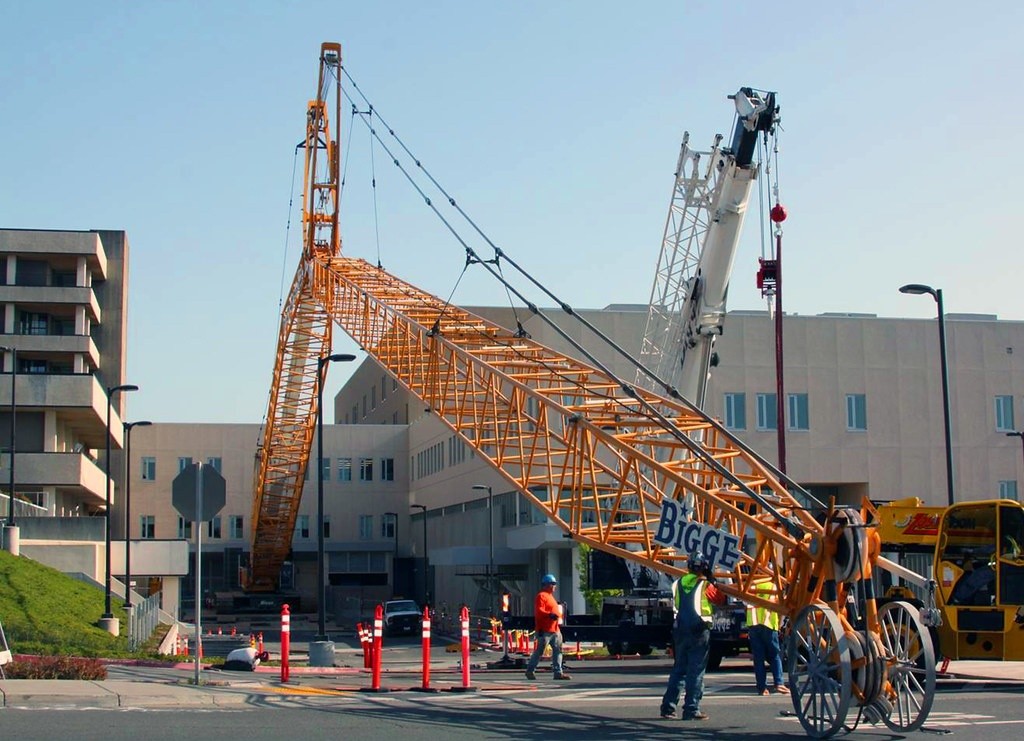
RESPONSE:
[549,613,558,620]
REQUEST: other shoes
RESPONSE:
[554,674,572,680]
[526,671,536,680]
[690,712,709,720]
[661,712,677,719]
[776,685,790,692]
[759,688,769,695]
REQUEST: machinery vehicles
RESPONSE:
[486,86,1023,683]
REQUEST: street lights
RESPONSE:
[471,484,495,617]
[384,512,398,599]
[0,343,17,526]
[314,354,356,641]
[100,383,140,618]
[899,284,955,507]
[123,420,153,609]
[412,504,429,606]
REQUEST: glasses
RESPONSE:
[546,582,556,585]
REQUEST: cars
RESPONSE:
[382,598,421,635]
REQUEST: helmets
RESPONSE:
[688,551,705,563]
[541,574,556,583]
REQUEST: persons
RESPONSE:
[659,550,725,721]
[743,560,792,697]
[523,574,572,681]
[1000,520,1024,557]
